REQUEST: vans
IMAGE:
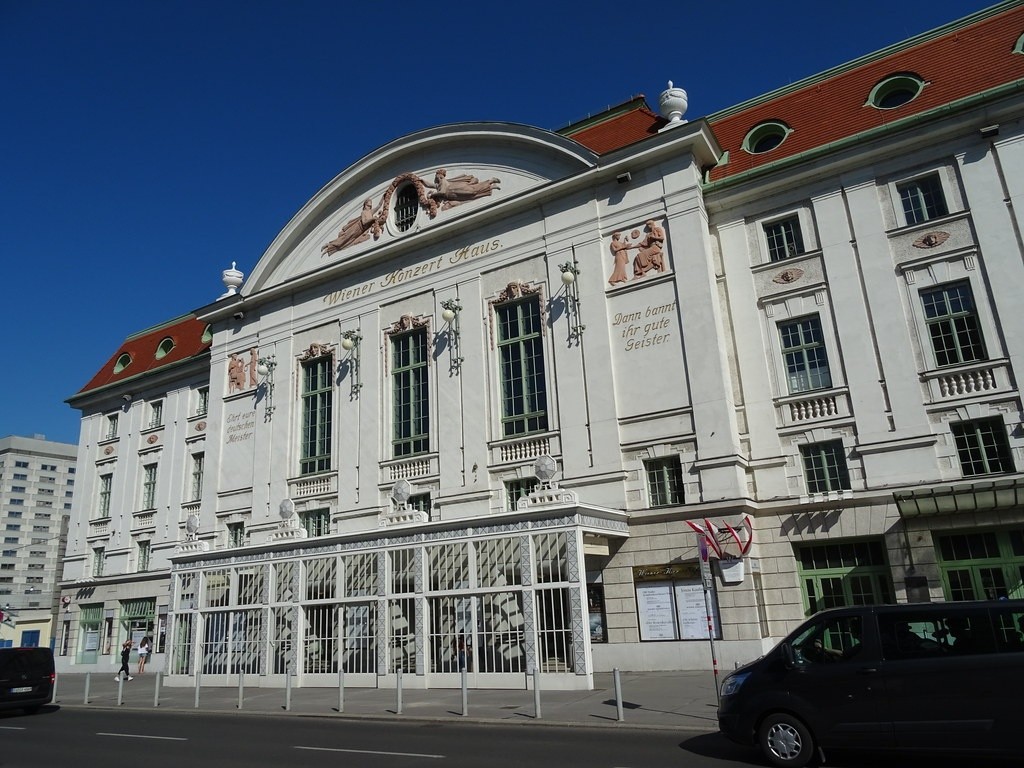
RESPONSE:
[715,598,1024,768]
[0,646,57,718]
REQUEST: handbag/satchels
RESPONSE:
[121,651,125,656]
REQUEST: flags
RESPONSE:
[685,516,752,558]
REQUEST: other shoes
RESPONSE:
[128,677,133,681]
[114,677,119,681]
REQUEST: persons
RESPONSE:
[507,282,521,300]
[137,637,150,674]
[320,193,385,258]
[814,618,863,659]
[400,315,413,331]
[945,616,971,648]
[308,343,320,357]
[608,220,665,286]
[227,348,257,392]
[416,169,501,211]
[114,639,134,682]
